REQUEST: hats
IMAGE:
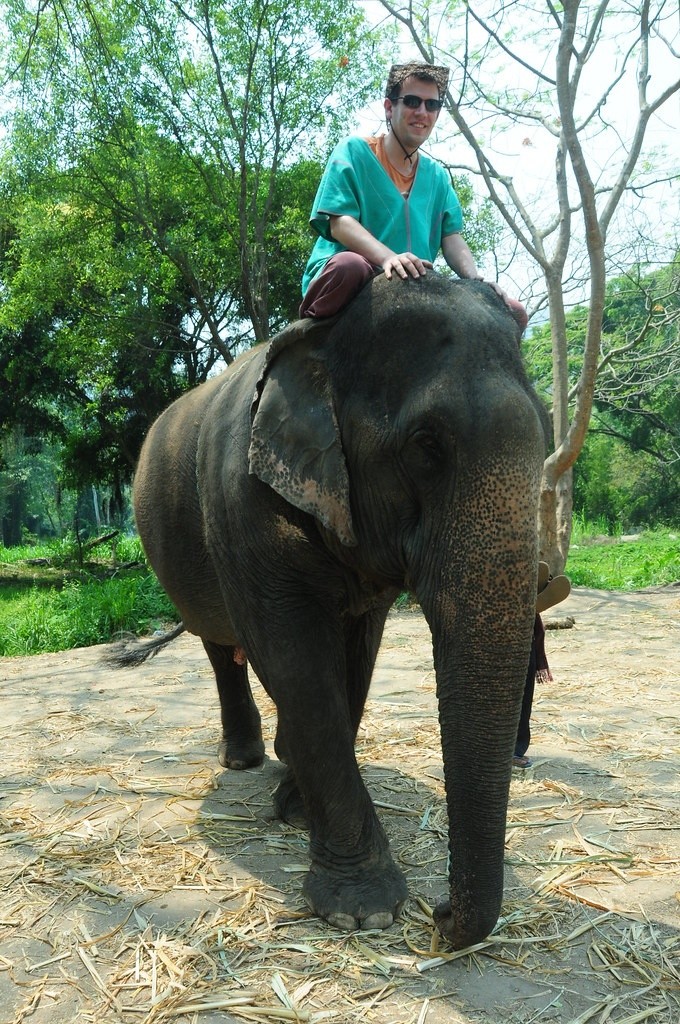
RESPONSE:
[387,60,449,107]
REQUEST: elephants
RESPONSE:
[131,262,553,948]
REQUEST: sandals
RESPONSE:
[512,756,532,768]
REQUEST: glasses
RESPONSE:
[392,95,443,111]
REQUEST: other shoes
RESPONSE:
[536,575,571,614]
[537,561,553,594]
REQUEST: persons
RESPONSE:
[299,62,528,334]
[512,613,545,768]
